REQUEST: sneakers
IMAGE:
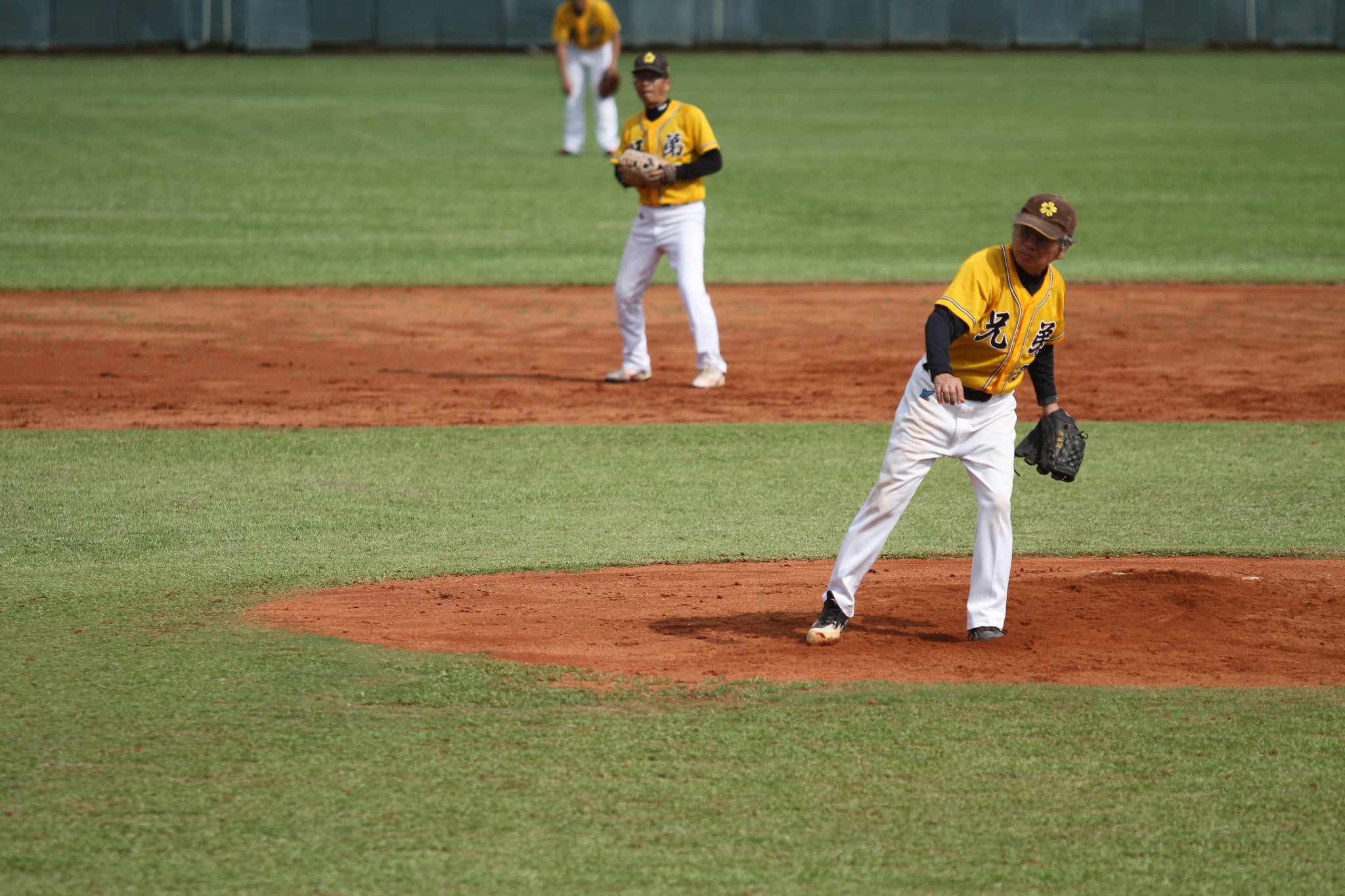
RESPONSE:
[692,369,726,389]
[806,590,851,646]
[968,623,1004,641]
[605,368,653,382]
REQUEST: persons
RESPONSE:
[552,0,622,157]
[807,194,1085,646]
[604,50,728,388]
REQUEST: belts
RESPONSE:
[924,362,993,403]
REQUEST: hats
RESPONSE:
[1014,193,1078,240]
[631,51,670,76]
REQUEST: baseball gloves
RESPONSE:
[617,148,677,188]
[599,66,620,98]
[1015,409,1085,482]
